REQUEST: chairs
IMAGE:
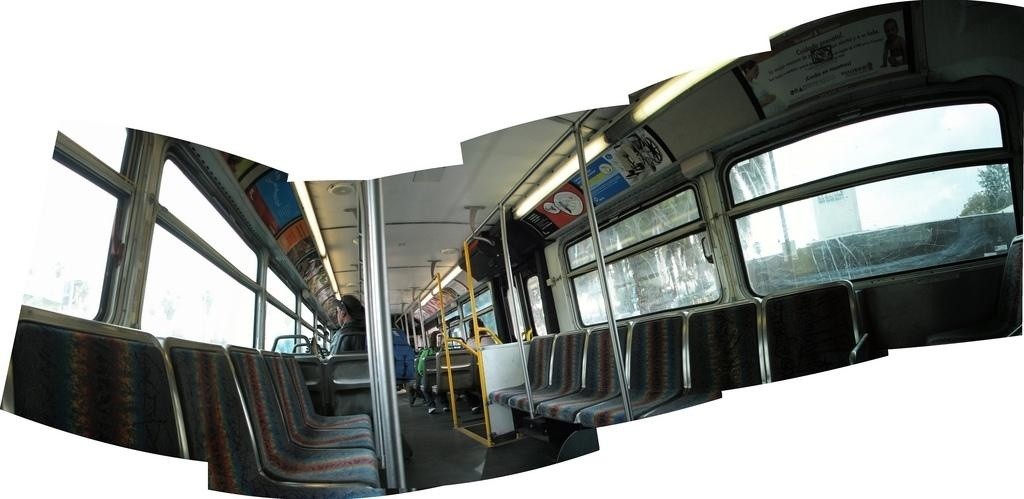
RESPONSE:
[414,336,497,394]
[489,280,869,439]
[0,305,387,499]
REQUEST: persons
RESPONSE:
[740,60,782,119]
[615,128,645,178]
[329,294,367,356]
[418,331,454,414]
[879,18,908,68]
[465,317,494,415]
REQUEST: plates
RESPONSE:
[552,191,583,216]
[542,202,560,214]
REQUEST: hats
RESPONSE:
[334,295,364,321]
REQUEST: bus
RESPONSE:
[712,212,1018,301]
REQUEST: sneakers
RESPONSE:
[428,408,435,414]
[442,407,448,411]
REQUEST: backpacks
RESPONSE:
[391,327,415,385]
